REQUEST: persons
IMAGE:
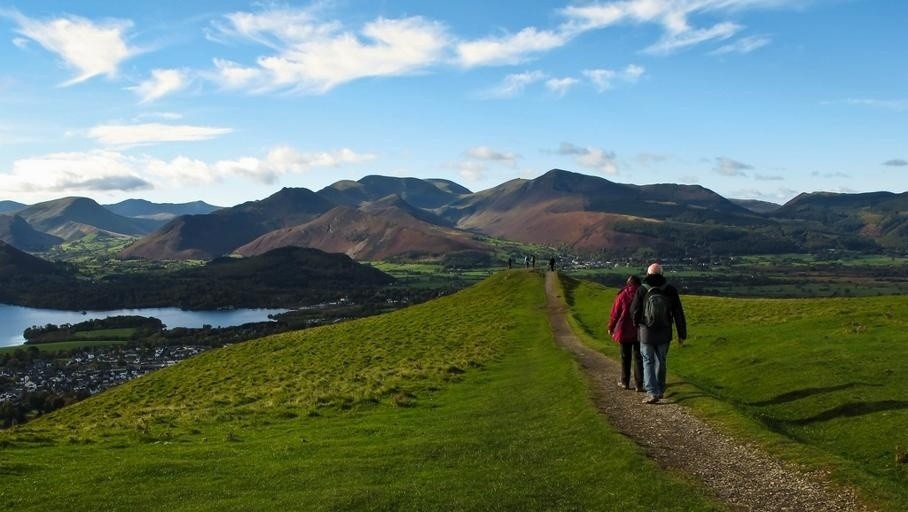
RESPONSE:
[548,255,556,272]
[507,257,513,269]
[524,255,531,269]
[630,263,688,404]
[530,254,536,268]
[608,276,646,394]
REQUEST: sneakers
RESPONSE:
[618,381,643,392]
[642,394,663,403]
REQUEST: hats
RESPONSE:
[648,264,662,275]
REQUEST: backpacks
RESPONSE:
[641,282,669,329]
